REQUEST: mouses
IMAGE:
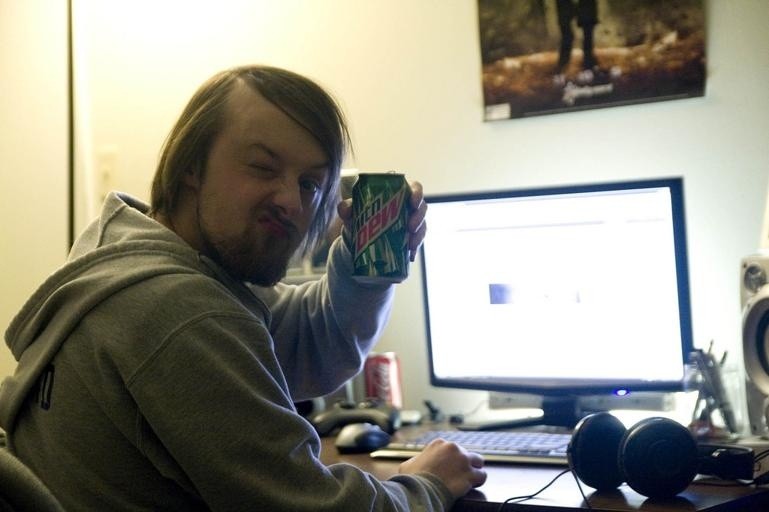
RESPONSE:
[334,423,392,455]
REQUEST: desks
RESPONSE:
[317,412,768,512]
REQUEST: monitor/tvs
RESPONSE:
[416,175,695,429]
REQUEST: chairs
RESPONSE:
[1,443,66,511]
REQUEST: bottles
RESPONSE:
[364,352,402,411]
[351,174,412,286]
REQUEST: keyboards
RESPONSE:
[369,428,573,465]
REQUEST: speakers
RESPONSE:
[739,252,768,441]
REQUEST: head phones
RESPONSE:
[565,411,756,502]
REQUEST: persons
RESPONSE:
[0,67,487,512]
